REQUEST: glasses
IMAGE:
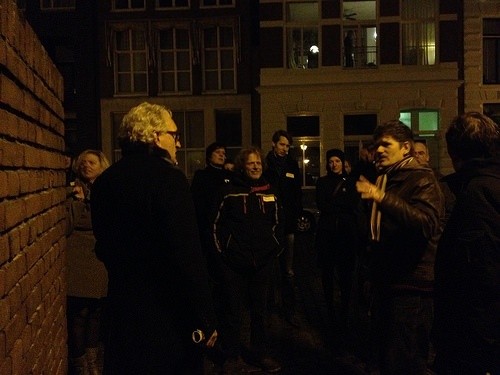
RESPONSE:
[166,131,182,140]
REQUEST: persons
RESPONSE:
[191,129,304,375]
[65,143,112,375]
[317,121,445,375]
[344,31,356,67]
[434,113,500,375]
[91,99,218,375]
